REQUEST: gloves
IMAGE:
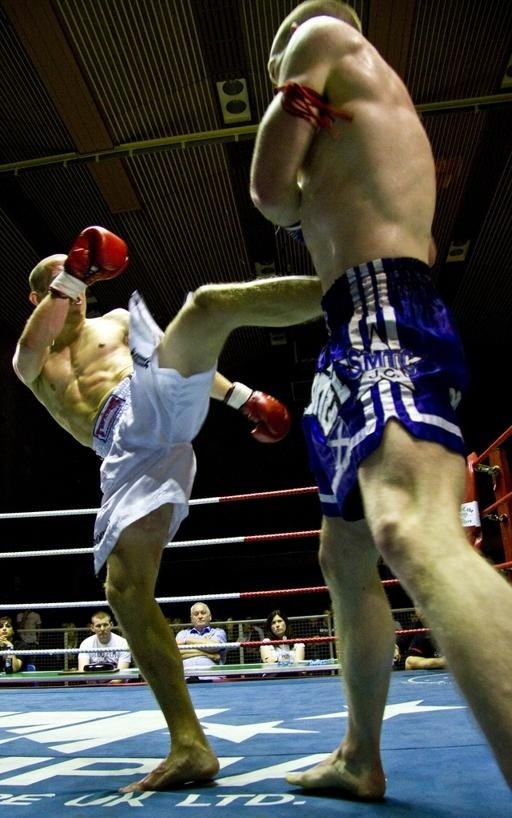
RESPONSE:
[47,226,130,305]
[223,381,291,444]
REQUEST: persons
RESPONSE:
[0,602,447,684]
[245,1,512,805]
[11,226,329,795]
